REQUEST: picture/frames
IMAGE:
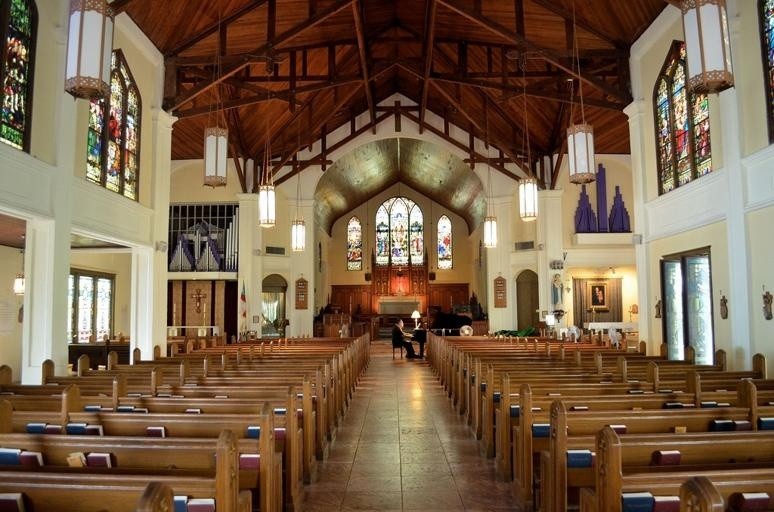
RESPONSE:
[586,282,609,311]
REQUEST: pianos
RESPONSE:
[411,312,472,358]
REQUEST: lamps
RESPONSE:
[201,0,306,253]
[63,2,114,100]
[481,1,597,250]
[679,0,736,98]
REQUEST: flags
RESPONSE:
[238,280,247,321]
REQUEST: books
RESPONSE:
[714,377,753,392]
[1,492,25,512]
[27,422,104,436]
[566,422,772,510]
[240,453,261,469]
[0,447,112,469]
[147,426,166,438]
[480,382,589,438]
[597,377,683,394]
[246,391,318,439]
[85,384,228,415]
[174,496,216,512]
[664,397,773,432]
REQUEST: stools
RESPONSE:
[392,345,402,360]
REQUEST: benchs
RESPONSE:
[425,329,774,511]
[0,330,372,512]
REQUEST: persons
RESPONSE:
[596,290,603,303]
[553,273,564,309]
[592,286,604,305]
[390,319,420,359]
